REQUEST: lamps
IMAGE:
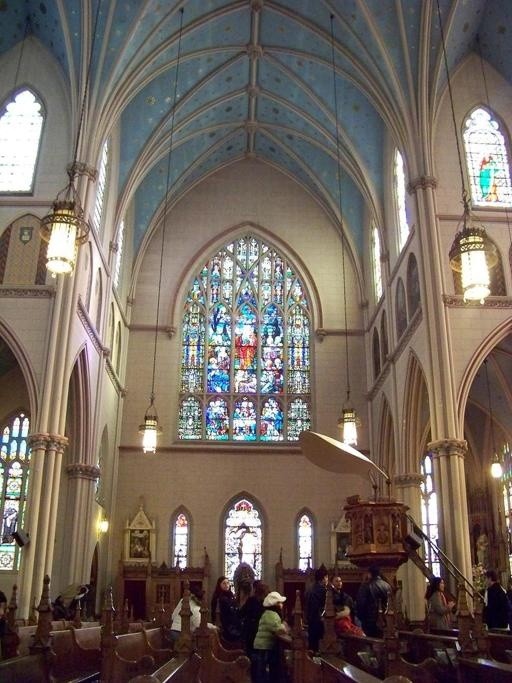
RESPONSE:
[140,6,189,454]
[479,356,503,478]
[36,0,103,274]
[326,8,361,447]
[427,1,502,305]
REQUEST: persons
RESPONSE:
[54,594,68,619]
[0,590,22,660]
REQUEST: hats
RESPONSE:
[263,591,287,606]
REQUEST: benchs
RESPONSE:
[1,572,512,681]
